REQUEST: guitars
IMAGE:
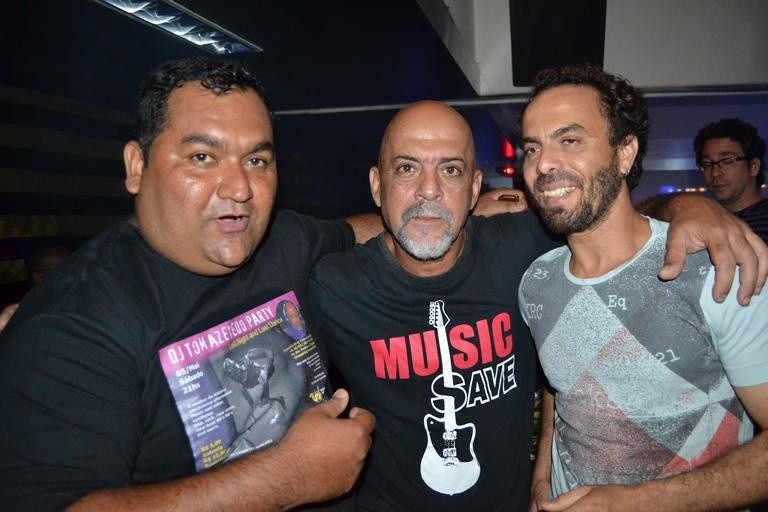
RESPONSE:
[420,298,481,495]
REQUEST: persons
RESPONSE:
[23,238,77,294]
[691,114,767,250]
[0,98,768,511]
[0,50,533,512]
[515,61,768,512]
[275,300,309,340]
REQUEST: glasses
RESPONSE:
[698,156,749,173]
